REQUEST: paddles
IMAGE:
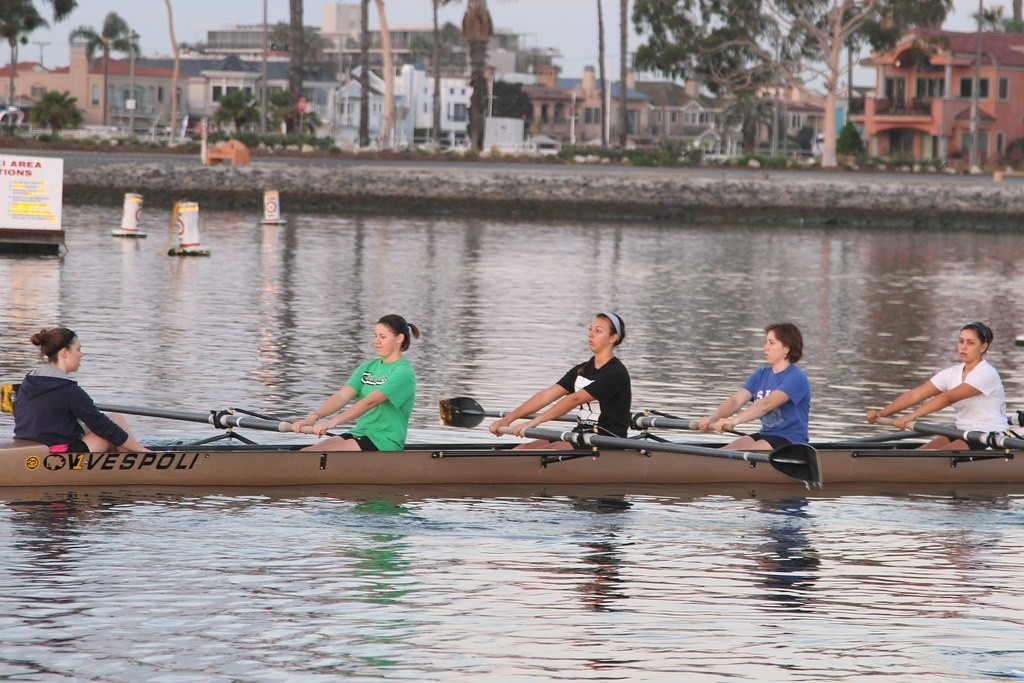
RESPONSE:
[0,383,328,437]
[496,425,821,485]
[874,416,1024,450]
[438,396,734,434]
[1007,409,1024,431]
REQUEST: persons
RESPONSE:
[488,312,631,449]
[866,321,1010,450]
[698,322,811,451]
[12,327,154,453]
[290,314,420,451]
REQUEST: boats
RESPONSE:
[0,431,1024,489]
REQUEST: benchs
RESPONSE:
[205,139,250,166]
[994,171,1024,183]
